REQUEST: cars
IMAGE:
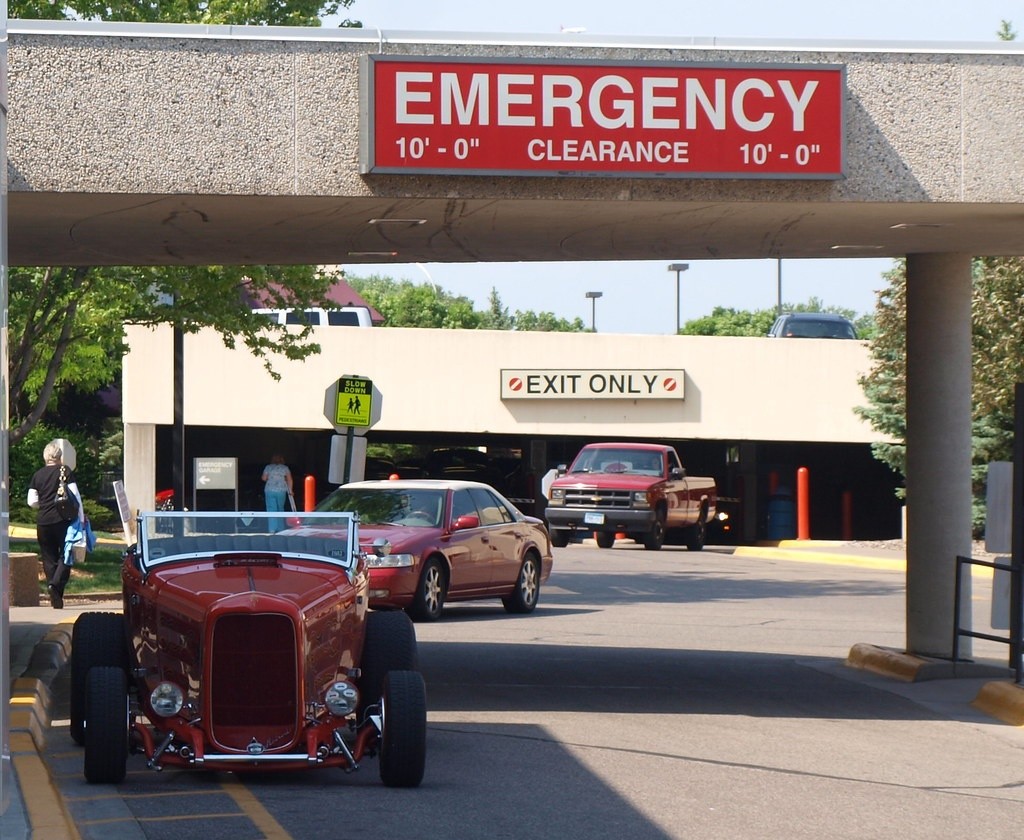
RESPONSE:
[69,510,428,790]
[274,478,554,626]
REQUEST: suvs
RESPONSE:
[768,311,859,340]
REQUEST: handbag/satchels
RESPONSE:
[73,531,87,562]
[54,467,80,521]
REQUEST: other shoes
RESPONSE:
[50,585,64,610]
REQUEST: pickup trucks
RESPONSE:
[545,443,717,548]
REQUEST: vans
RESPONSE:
[244,306,375,328]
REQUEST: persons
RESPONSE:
[261,450,294,534]
[27,442,86,610]
